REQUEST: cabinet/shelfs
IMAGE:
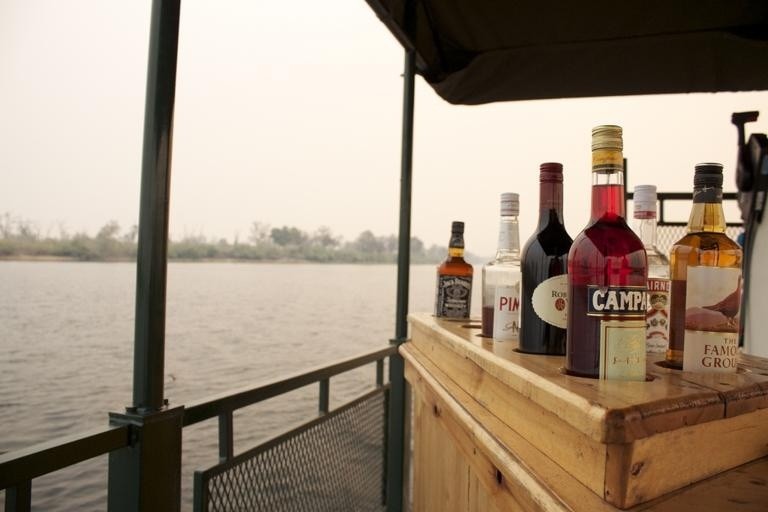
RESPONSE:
[398,315,768,512]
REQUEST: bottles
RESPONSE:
[668,161,744,376]
[436,221,473,319]
[633,185,670,354]
[567,124,648,381]
[518,162,573,356]
[483,192,525,338]
